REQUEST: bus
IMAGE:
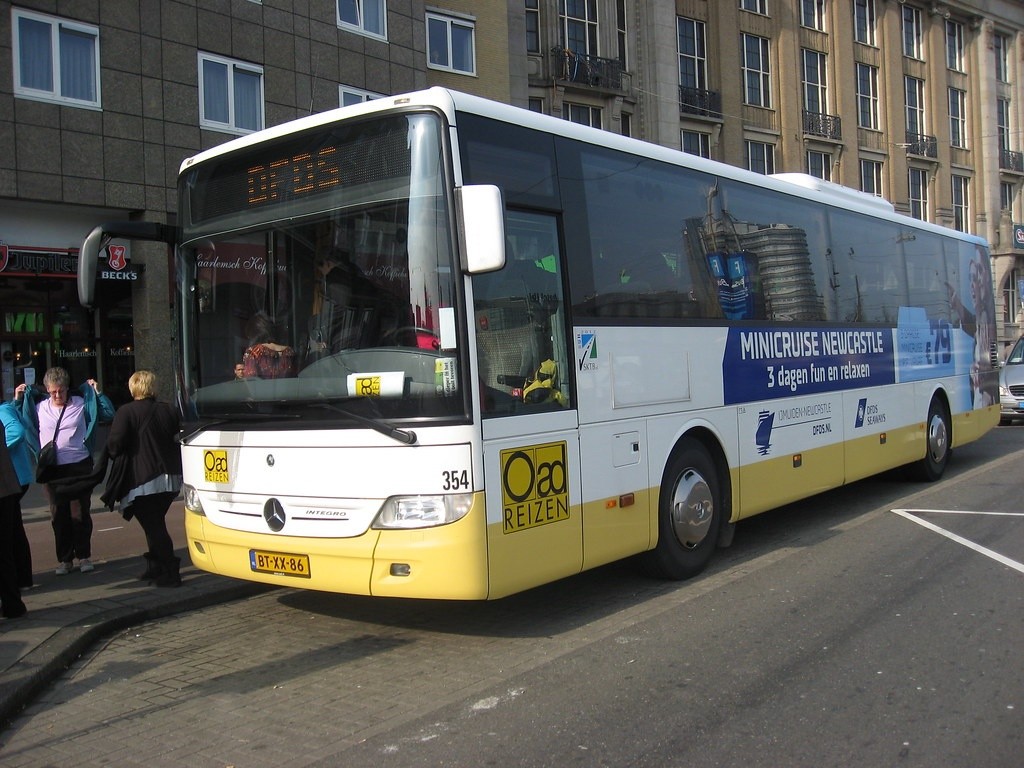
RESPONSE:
[79,86,1003,600]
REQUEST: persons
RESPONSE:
[100,370,183,587]
[11,367,116,575]
[945,243,999,409]
[235,312,297,379]
[306,244,380,363]
[0,399,33,617]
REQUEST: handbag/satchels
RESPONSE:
[36,395,70,483]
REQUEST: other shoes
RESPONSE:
[0,609,7,619]
[19,586,33,591]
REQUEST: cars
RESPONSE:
[998,332,1024,426]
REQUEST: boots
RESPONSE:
[141,553,181,587]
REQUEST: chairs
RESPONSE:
[477,257,952,393]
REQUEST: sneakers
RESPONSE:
[55,561,74,575]
[79,558,94,573]
